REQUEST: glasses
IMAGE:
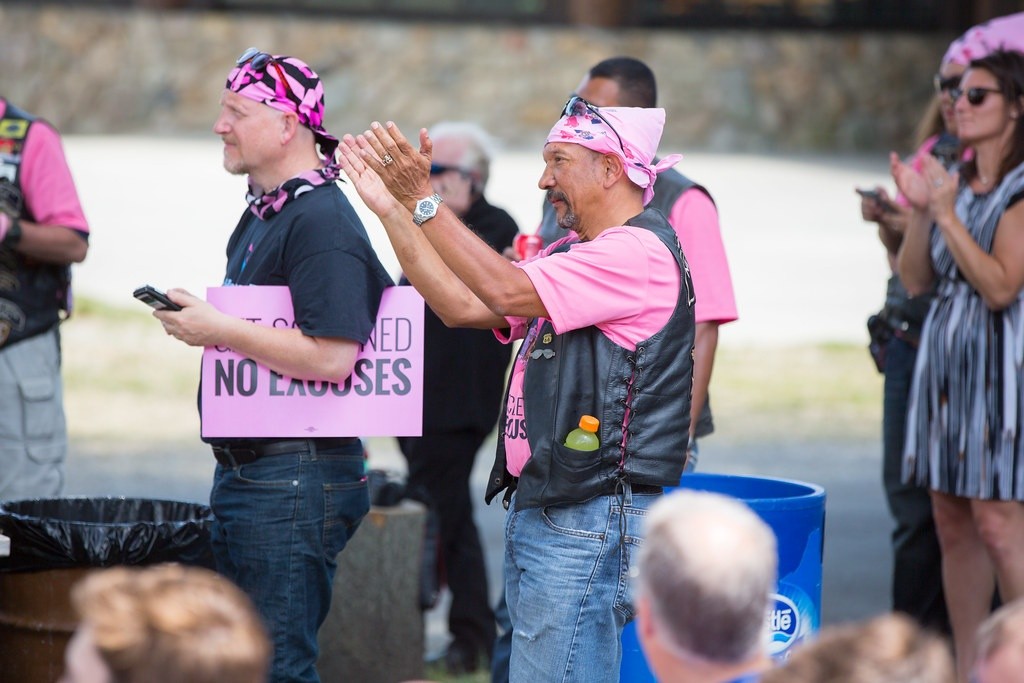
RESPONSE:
[235,48,294,100]
[560,95,626,155]
[933,75,962,92]
[430,163,465,174]
[954,87,1004,104]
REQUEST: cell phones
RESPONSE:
[134,287,182,311]
[855,188,899,214]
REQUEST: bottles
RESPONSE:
[566,414,601,451]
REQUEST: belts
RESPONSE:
[213,437,358,467]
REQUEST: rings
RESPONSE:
[383,154,393,165]
[934,177,942,187]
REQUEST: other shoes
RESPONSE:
[422,626,495,682]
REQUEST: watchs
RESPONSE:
[4,222,22,249]
[413,194,443,226]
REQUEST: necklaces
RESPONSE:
[982,177,987,183]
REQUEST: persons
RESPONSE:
[772,615,953,683]
[152,46,395,683]
[862,15,1024,683]
[0,96,89,502]
[396,122,520,674]
[65,564,272,683]
[633,487,779,683]
[494,57,738,683]
[339,94,695,683]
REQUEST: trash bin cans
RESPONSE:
[1,496,215,683]
